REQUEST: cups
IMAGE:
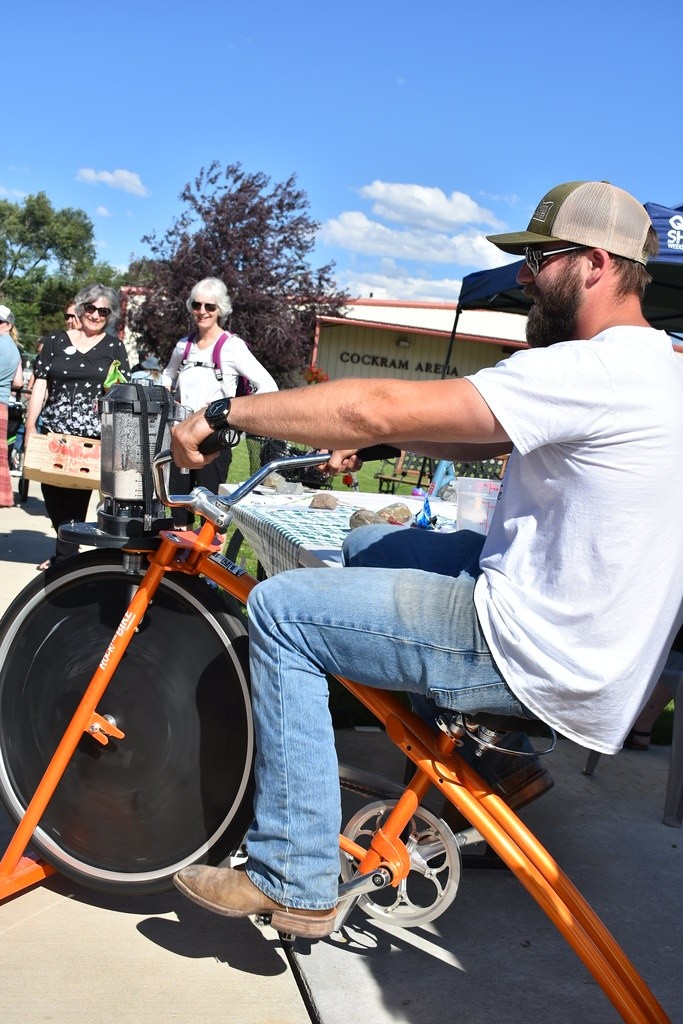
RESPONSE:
[456,477,502,534]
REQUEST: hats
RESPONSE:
[141,358,160,370]
[485,180,653,265]
[0,304,15,325]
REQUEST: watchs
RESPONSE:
[204,397,237,429]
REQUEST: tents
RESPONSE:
[415,201,683,490]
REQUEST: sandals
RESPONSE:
[626,730,651,750]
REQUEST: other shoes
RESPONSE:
[173,864,338,939]
[443,760,555,835]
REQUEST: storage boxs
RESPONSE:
[25,433,102,490]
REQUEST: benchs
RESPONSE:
[376,449,510,494]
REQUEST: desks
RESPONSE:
[219,484,457,570]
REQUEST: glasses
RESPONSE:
[191,302,218,312]
[523,246,585,277]
[82,302,111,317]
[64,313,75,320]
[0,320,7,325]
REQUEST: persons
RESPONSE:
[621,627,683,751]
[24,282,132,574]
[15,341,45,506]
[0,304,26,507]
[154,275,279,593]
[65,302,76,330]
[169,176,683,940]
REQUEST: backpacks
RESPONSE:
[181,330,252,397]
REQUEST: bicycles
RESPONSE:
[0,429,673,1024]
